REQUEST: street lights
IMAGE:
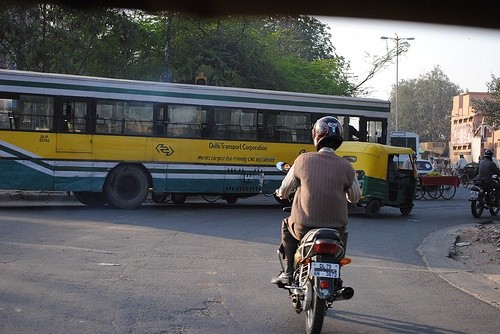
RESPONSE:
[381,35,414,132]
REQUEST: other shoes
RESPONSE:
[270,270,292,283]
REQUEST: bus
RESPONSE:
[391,131,420,168]
[0,69,391,210]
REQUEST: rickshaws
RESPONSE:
[415,175,460,200]
[455,165,480,188]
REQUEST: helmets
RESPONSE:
[312,116,344,152]
[484,149,493,156]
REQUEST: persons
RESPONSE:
[475,149,499,204]
[454,155,467,178]
[341,116,360,141]
[433,159,440,172]
[442,160,453,174]
[270,116,362,285]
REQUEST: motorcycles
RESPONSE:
[271,161,355,334]
[333,141,419,216]
[468,175,500,218]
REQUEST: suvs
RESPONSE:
[416,160,435,177]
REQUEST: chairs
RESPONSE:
[8,108,311,142]
